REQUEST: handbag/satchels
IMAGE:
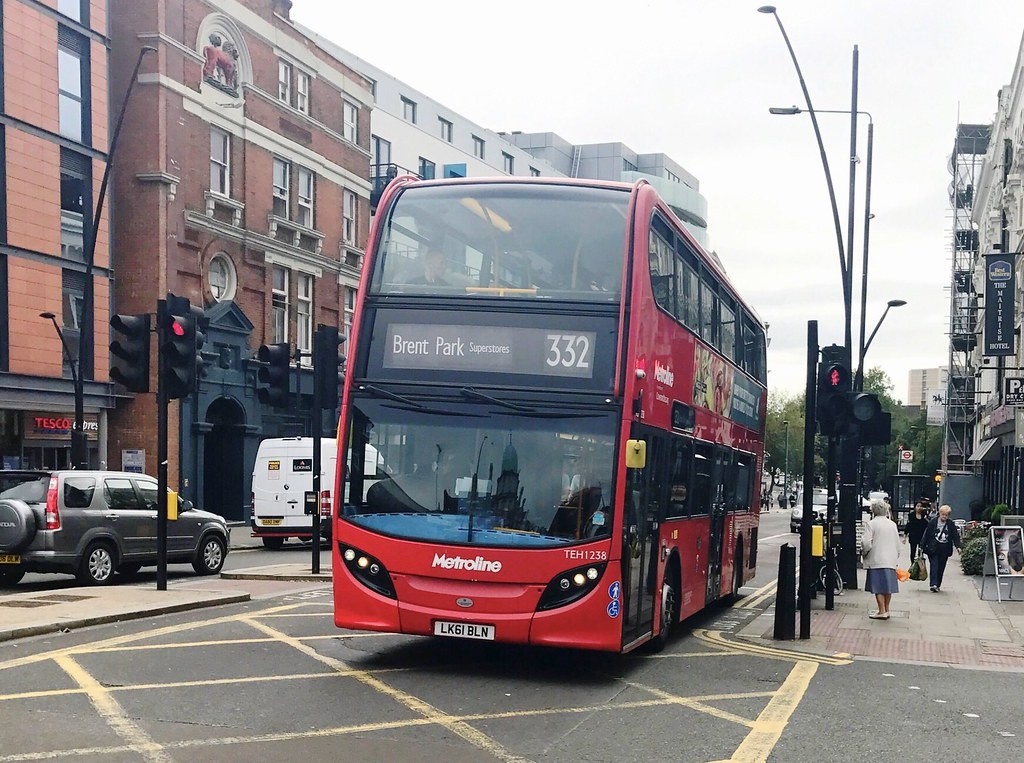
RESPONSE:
[897,569,911,582]
[925,538,940,559]
[908,550,928,581]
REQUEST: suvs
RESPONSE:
[0,471,231,586]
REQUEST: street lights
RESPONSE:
[755,4,909,591]
[783,421,789,508]
[38,46,160,471]
[910,425,928,472]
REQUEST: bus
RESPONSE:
[331,175,768,656]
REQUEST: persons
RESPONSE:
[920,499,939,522]
[919,504,963,593]
[760,489,770,511]
[884,497,893,520]
[902,502,929,565]
[861,501,900,619]
[777,492,784,507]
[407,249,449,286]
[789,491,798,508]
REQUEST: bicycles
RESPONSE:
[817,550,845,595]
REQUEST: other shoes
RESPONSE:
[869,611,891,620]
[929,585,940,592]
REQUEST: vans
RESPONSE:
[248,436,392,549]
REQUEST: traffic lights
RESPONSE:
[818,343,851,435]
[257,343,291,411]
[846,392,890,445]
[107,313,151,394]
[312,322,349,409]
[156,292,207,401]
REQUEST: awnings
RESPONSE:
[967,436,1003,460]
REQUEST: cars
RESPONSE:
[790,489,888,533]
